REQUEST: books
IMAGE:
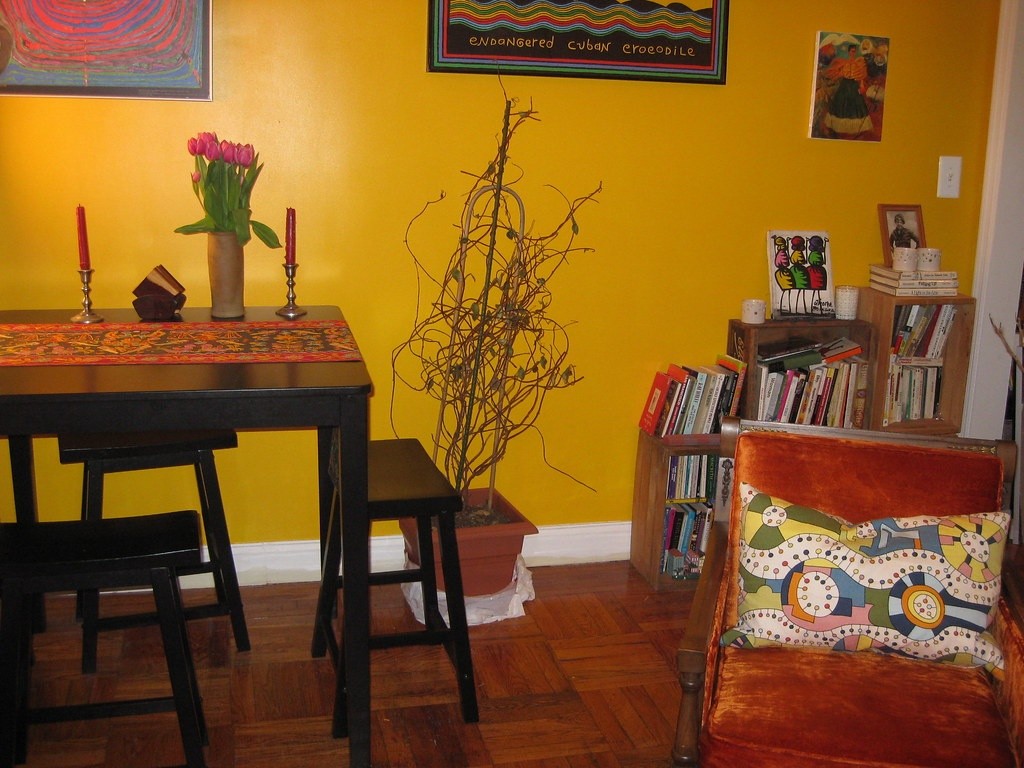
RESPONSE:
[883,305,957,426]
[865,261,959,297]
[755,336,868,431]
[660,454,720,570]
[638,353,747,438]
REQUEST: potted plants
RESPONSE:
[387,59,603,597]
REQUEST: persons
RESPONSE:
[889,213,920,253]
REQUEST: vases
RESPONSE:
[207,230,246,319]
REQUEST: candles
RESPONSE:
[75,203,91,269]
[284,206,297,265]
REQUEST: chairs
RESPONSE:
[669,415,1024,768]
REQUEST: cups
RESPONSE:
[917,248,942,272]
[835,284,859,320]
[741,299,767,324]
[892,247,918,273]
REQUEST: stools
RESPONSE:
[310,437,479,740]
[0,510,210,768]
[55,429,251,674]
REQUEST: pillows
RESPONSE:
[717,479,1014,682]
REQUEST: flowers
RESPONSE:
[173,132,284,249]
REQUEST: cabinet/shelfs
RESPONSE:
[629,286,977,592]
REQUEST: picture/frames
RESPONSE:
[876,202,928,268]
[426,0,730,85]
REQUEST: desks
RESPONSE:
[0,306,375,768]
